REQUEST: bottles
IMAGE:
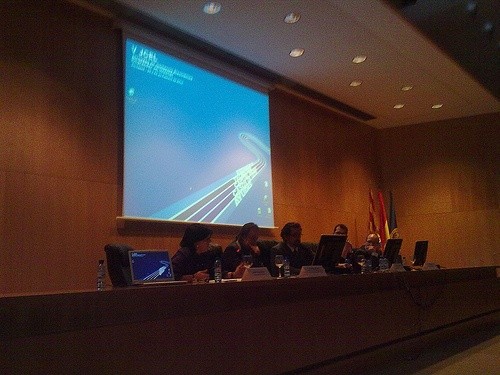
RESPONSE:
[214,257,222,284]
[96,259,105,291]
[379,256,384,270]
[284,256,291,278]
[384,256,390,271]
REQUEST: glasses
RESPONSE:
[366,241,378,243]
[334,232,346,235]
[199,237,211,242]
[286,233,303,238]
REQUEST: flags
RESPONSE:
[367,188,379,234]
[376,190,391,252]
[389,189,402,239]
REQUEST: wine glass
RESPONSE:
[275,255,284,279]
[408,255,416,271]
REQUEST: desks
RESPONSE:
[0,264,500,375]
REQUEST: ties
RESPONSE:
[294,249,297,255]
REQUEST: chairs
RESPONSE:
[105,241,360,286]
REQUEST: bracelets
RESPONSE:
[192,273,195,279]
[230,271,234,278]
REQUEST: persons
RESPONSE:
[222,221,282,277]
[171,224,251,282]
[333,224,354,269]
[354,232,384,271]
[268,222,316,276]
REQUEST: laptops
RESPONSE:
[411,241,429,266]
[311,234,347,271]
[128,249,188,285]
[383,239,403,263]
